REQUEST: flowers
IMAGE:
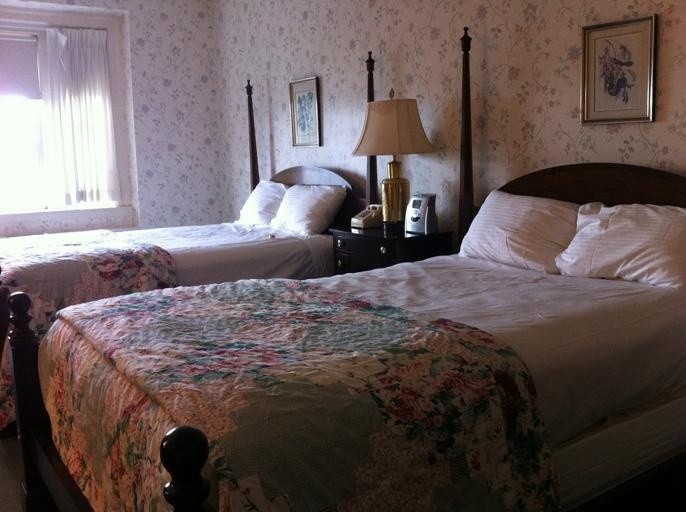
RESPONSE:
[597,37,638,105]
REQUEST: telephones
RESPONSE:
[350,204,382,228]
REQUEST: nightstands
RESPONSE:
[327,224,458,277]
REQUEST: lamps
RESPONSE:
[351,85,446,227]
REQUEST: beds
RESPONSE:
[0,46,385,437]
[4,21,685,510]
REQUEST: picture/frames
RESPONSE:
[575,12,661,129]
[286,73,325,149]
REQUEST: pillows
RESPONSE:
[552,197,686,290]
[456,184,577,275]
[269,179,348,239]
[232,177,288,229]
